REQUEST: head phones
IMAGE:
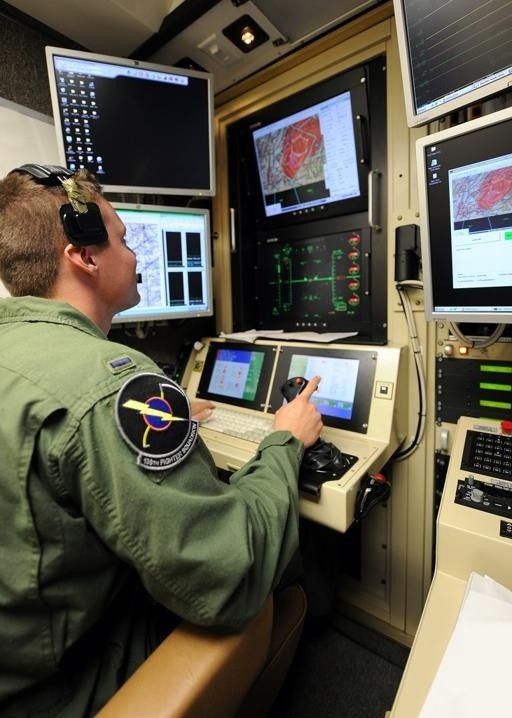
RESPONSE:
[8,164,108,246]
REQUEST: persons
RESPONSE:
[1,164,326,718]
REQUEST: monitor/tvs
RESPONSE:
[233,67,372,229]
[45,45,217,197]
[416,106,512,324]
[194,341,277,413]
[269,346,378,434]
[393,0,512,128]
[240,227,370,332]
[106,201,214,324]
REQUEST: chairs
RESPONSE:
[93,558,308,715]
